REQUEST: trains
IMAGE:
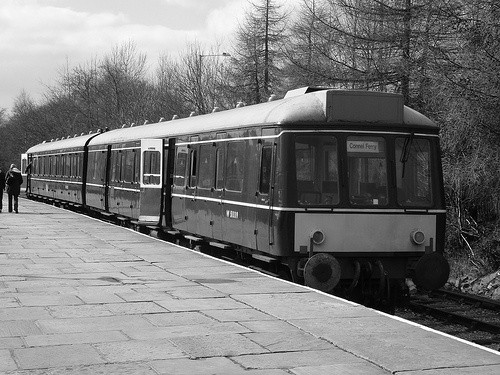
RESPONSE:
[23,85,452,296]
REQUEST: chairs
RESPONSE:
[299,179,376,205]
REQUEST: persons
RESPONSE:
[5,163,23,213]
[0,169,5,212]
[24,162,31,174]
